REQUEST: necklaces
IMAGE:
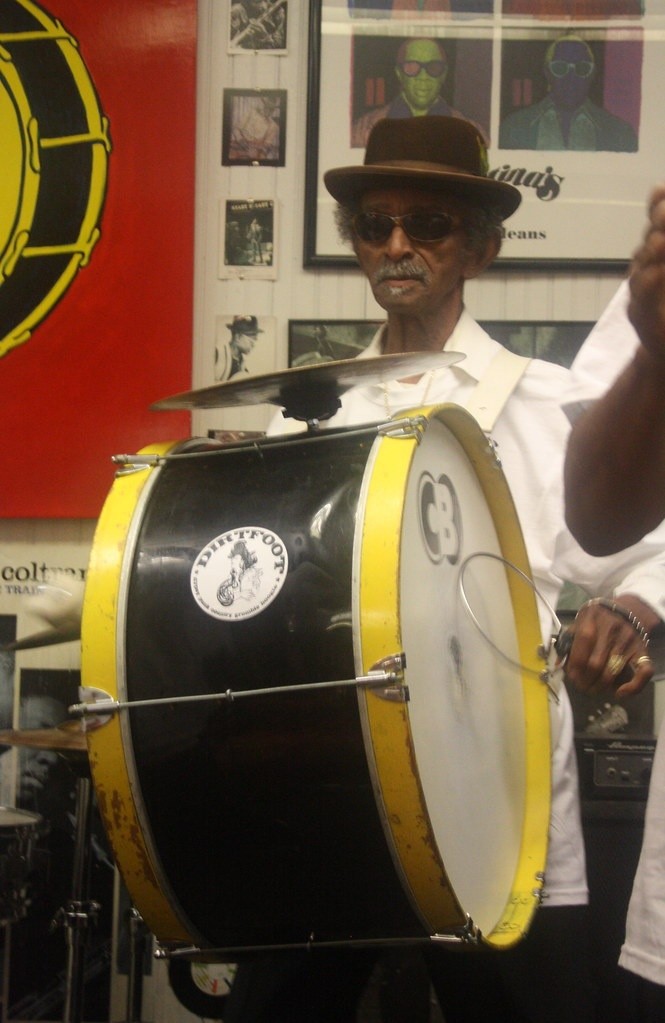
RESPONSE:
[383,372,433,418]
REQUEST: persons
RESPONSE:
[350,36,638,153]
[211,1,295,385]
[322,113,665,1023]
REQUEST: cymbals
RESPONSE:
[149,348,468,409]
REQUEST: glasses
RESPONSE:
[348,208,468,244]
[549,60,596,79]
[398,59,447,78]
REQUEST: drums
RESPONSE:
[69,403,551,952]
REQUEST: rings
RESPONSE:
[606,654,626,676]
[631,655,654,671]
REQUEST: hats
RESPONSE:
[323,115,523,224]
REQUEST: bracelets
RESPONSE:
[575,596,651,651]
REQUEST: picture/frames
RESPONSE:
[285,315,598,372]
[216,193,279,284]
[300,0,665,277]
[225,0,290,58]
[220,86,290,169]
